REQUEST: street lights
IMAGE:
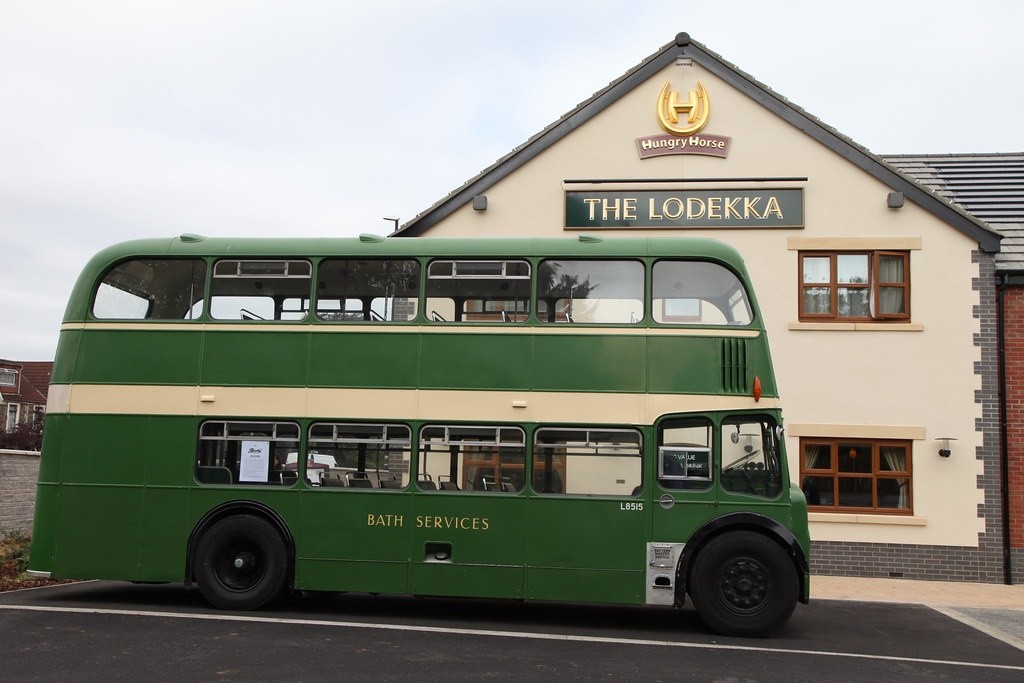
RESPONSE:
[383,216,398,230]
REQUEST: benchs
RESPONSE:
[501,309,512,322]
[418,474,437,490]
[367,309,386,322]
[240,309,265,320]
[319,472,344,487]
[482,475,496,492]
[438,475,457,491]
[502,477,516,492]
[279,472,298,485]
[345,471,373,488]
[377,471,401,489]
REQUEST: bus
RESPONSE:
[24,233,811,635]
